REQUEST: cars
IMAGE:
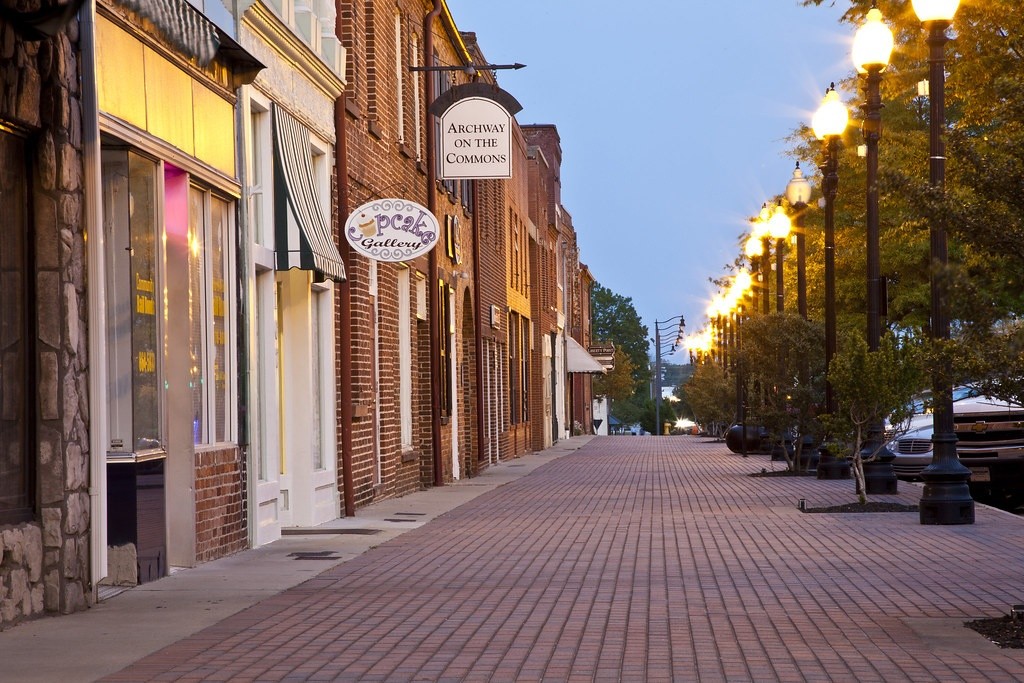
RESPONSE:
[881,373,1024,514]
[631,424,645,435]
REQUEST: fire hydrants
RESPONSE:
[662,418,671,436]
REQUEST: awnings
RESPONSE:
[566,336,608,375]
[274,103,345,283]
[609,414,621,424]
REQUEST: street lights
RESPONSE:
[656,314,686,435]
[910,0,975,525]
[766,198,796,462]
[852,8,898,496]
[783,161,821,470]
[744,235,763,423]
[810,80,853,480]
[688,268,752,378]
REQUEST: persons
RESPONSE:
[640,428,645,435]
[631,425,636,435]
